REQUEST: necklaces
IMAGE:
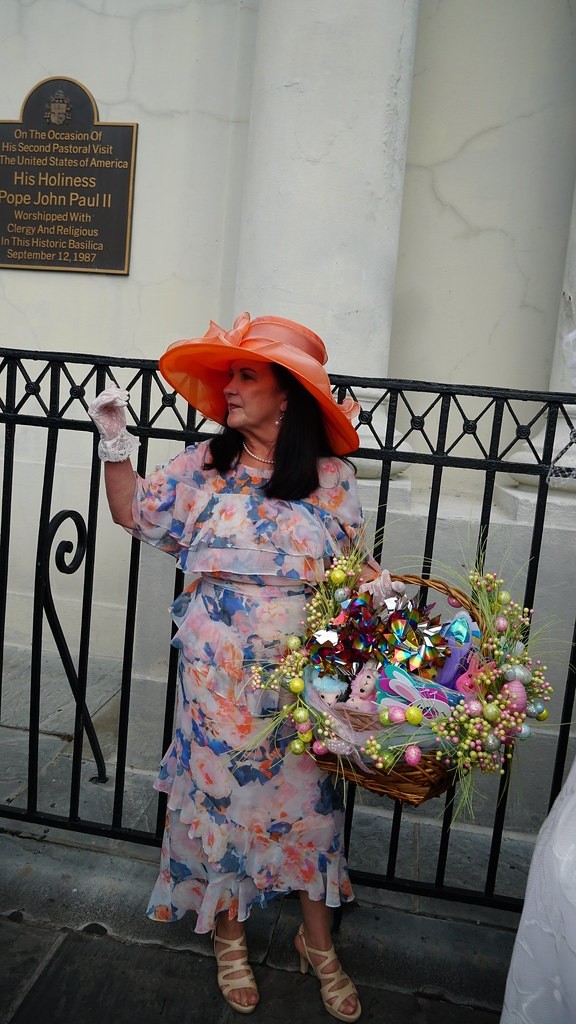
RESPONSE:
[243,442,276,464]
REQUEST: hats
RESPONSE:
[158,311,361,456]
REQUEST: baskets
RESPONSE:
[311,574,494,805]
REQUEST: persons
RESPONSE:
[89,311,413,1022]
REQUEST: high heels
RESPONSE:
[293,921,362,1023]
[210,913,260,1014]
